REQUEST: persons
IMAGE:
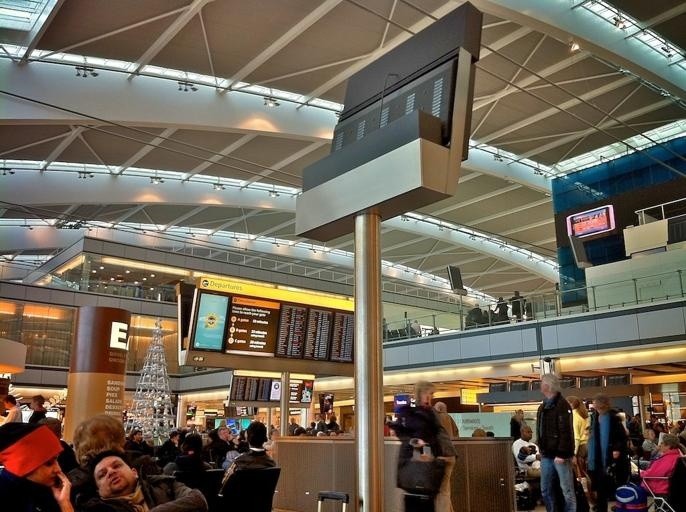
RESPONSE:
[465,304,493,327]
[411,320,421,337]
[382,319,389,331]
[431,328,440,334]
[508,291,526,322]
[493,297,510,324]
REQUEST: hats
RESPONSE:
[1,422,64,477]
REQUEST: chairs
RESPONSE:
[382,327,417,342]
[513,437,684,512]
[59,437,283,511]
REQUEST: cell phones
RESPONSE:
[566,205,616,239]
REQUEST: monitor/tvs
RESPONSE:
[447,265,467,296]
[294,1,483,243]
[178,281,355,408]
[215,419,251,435]
[394,394,410,412]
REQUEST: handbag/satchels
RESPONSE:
[575,462,593,501]
[614,482,645,505]
[394,447,449,496]
